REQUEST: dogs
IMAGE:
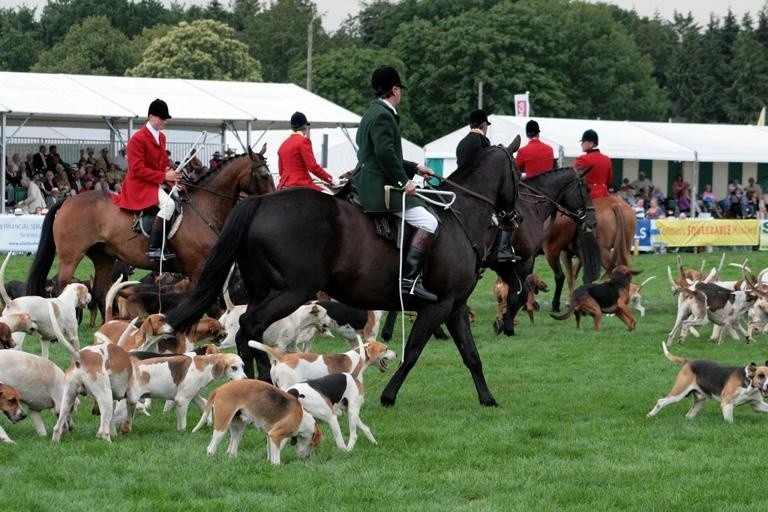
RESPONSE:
[1,250,399,465]
[666,252,767,346]
[591,275,656,317]
[409,303,476,324]
[493,273,550,326]
[549,261,644,331]
[646,341,767,425]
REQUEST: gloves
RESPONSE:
[330,174,342,186]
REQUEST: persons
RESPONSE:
[515,121,554,178]
[110,99,182,261]
[351,65,438,300]
[6,146,235,215]
[276,112,327,193]
[456,110,522,262]
[573,130,614,201]
[609,172,768,219]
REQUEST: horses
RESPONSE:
[27,142,276,325]
[381,165,596,343]
[538,206,604,313]
[157,133,533,408]
[561,196,636,307]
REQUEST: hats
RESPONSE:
[469,109,492,129]
[148,98,172,120]
[526,120,541,137]
[70,163,79,171]
[290,111,311,129]
[371,64,407,97]
[579,128,598,146]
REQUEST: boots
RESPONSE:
[147,215,179,262]
[491,227,525,264]
[398,226,440,304]
